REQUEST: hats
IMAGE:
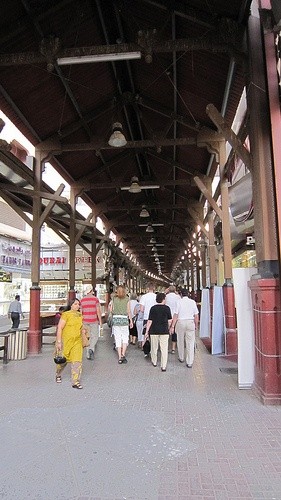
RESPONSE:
[85,286,92,293]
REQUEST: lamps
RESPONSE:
[56,51,141,65]
[151,253,165,275]
[138,223,163,226]
[146,223,153,232]
[152,247,158,251]
[150,237,156,243]
[107,120,127,148]
[139,206,150,217]
[146,244,165,246]
[121,185,161,190]
[128,176,140,194]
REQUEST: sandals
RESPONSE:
[56,375,62,382]
[72,381,83,389]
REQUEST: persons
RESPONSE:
[107,284,200,372]
[8,295,24,328]
[54,298,90,389]
[55,307,65,319]
[79,286,102,360]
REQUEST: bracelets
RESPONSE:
[55,341,62,343]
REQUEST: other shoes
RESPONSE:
[130,342,135,345]
[187,364,192,368]
[152,362,157,367]
[138,345,141,348]
[89,349,94,360]
[161,366,166,372]
[171,351,175,354]
[141,348,143,351]
[113,345,116,350]
[87,356,90,359]
[118,360,123,364]
[121,356,128,364]
[178,358,184,363]
[144,355,147,358]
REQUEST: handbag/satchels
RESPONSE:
[52,347,66,364]
[143,338,150,354]
[80,326,90,347]
